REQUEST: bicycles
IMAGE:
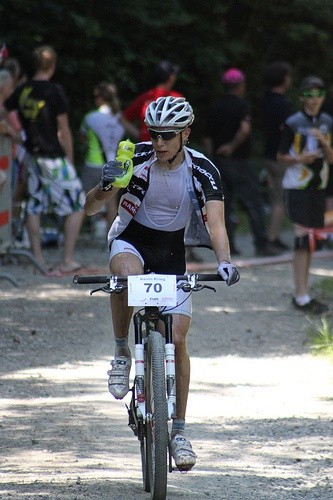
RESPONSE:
[73,273,236,499]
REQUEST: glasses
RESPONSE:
[147,127,186,141]
[300,90,327,100]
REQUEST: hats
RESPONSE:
[298,77,326,89]
[222,68,246,87]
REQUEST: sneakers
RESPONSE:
[170,433,197,466]
[107,350,132,399]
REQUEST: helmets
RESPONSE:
[144,95,194,129]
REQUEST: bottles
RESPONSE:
[111,139,135,188]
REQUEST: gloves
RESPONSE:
[217,260,241,286]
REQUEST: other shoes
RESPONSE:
[254,235,289,258]
[290,296,330,317]
[60,262,86,273]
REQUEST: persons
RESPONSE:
[278,78,333,313]
[84,97,240,468]
[260,62,321,251]
[1,46,89,274]
[203,68,278,256]
[79,80,130,226]
[118,61,183,141]
